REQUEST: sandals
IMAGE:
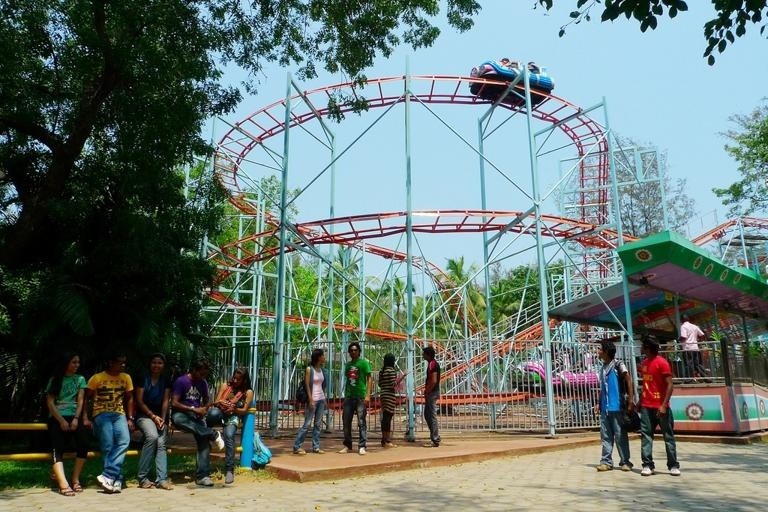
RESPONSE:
[59,483,82,496]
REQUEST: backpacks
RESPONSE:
[252,432,272,464]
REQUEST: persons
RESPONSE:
[495,57,541,75]
[169,357,225,486]
[41,348,89,497]
[135,352,174,490]
[634,340,768,383]
[81,350,137,493]
[291,348,329,455]
[421,346,441,447]
[594,339,635,470]
[377,352,413,448]
[638,334,681,476]
[337,341,372,455]
[532,341,601,380]
[206,364,254,483]
[677,312,706,383]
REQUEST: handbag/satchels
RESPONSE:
[296,380,308,401]
[621,411,641,432]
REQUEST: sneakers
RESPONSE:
[97,474,122,493]
[215,431,224,450]
[641,466,654,476]
[142,479,174,490]
[383,442,397,447]
[671,468,680,475]
[225,471,233,483]
[423,441,439,447]
[336,446,366,455]
[294,447,325,454]
[197,477,214,486]
[597,464,632,471]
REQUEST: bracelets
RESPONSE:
[129,415,135,420]
[73,417,79,419]
[190,405,195,413]
[147,411,157,419]
